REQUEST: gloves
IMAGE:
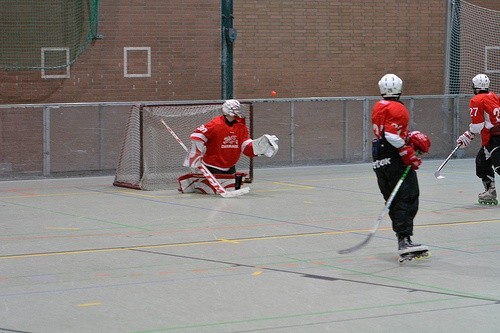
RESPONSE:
[405,131,431,154]
[456,130,475,149]
[398,145,422,173]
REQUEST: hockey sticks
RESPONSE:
[160,118,250,198]
[336,148,421,255]
[434,142,463,181]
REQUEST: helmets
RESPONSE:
[471,73,490,91]
[221,99,240,116]
[377,73,403,98]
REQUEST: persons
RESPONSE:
[178,100,280,195]
[457,73,500,205]
[372,74,431,263]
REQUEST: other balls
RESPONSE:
[271,90,278,97]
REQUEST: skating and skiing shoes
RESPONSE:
[477,182,499,206]
[396,235,431,264]
